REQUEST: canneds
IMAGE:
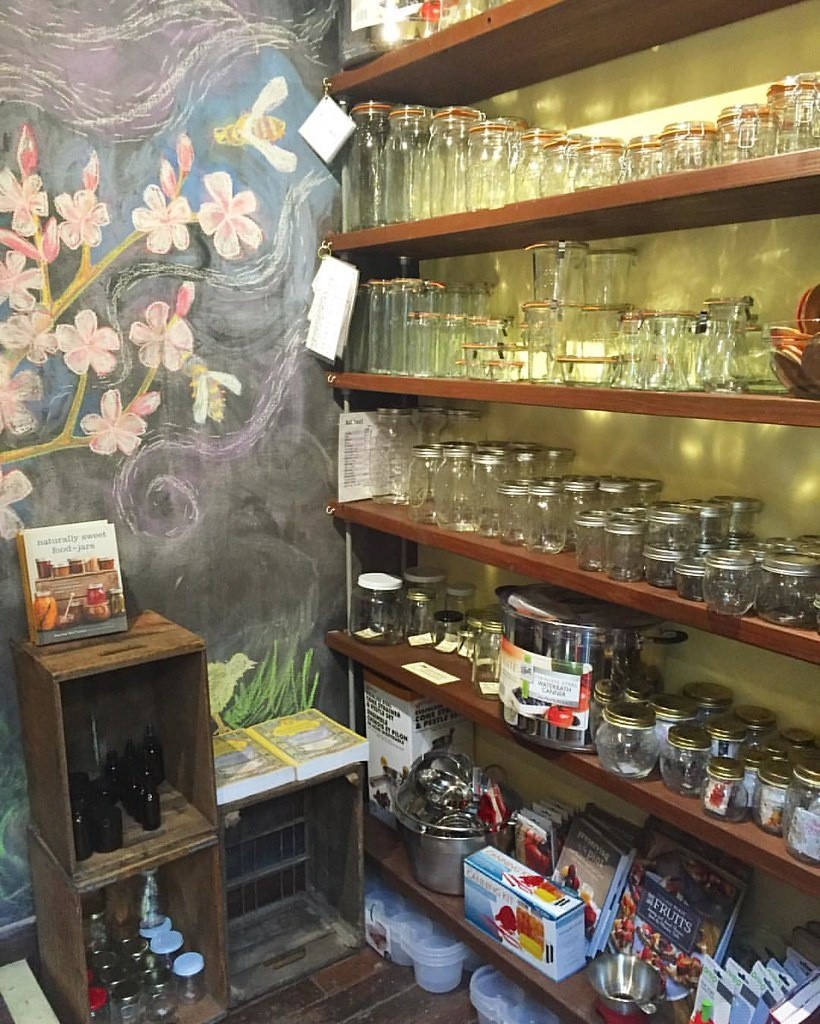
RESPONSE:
[32,558,126,631]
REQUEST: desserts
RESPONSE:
[554,864,602,934]
[610,891,703,987]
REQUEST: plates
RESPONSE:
[769,284,820,394]
[608,916,706,1001]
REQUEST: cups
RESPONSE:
[587,954,663,1015]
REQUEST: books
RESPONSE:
[208,728,297,806]
[14,520,128,646]
[247,706,371,782]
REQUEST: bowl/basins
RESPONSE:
[761,318,820,400]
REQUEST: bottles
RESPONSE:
[344,73,820,231]
[349,564,503,702]
[33,556,125,632]
[338,0,507,70]
[589,665,820,866]
[79,867,208,1024]
[69,723,165,862]
[341,239,785,396]
[516,907,544,961]
[370,404,820,634]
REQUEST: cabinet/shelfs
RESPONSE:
[8,605,227,1024]
[320,0,820,1024]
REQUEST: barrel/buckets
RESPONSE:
[393,760,523,896]
[494,584,688,751]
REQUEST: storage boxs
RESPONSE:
[463,843,586,982]
[468,962,561,1024]
[362,869,490,995]
[361,665,476,831]
